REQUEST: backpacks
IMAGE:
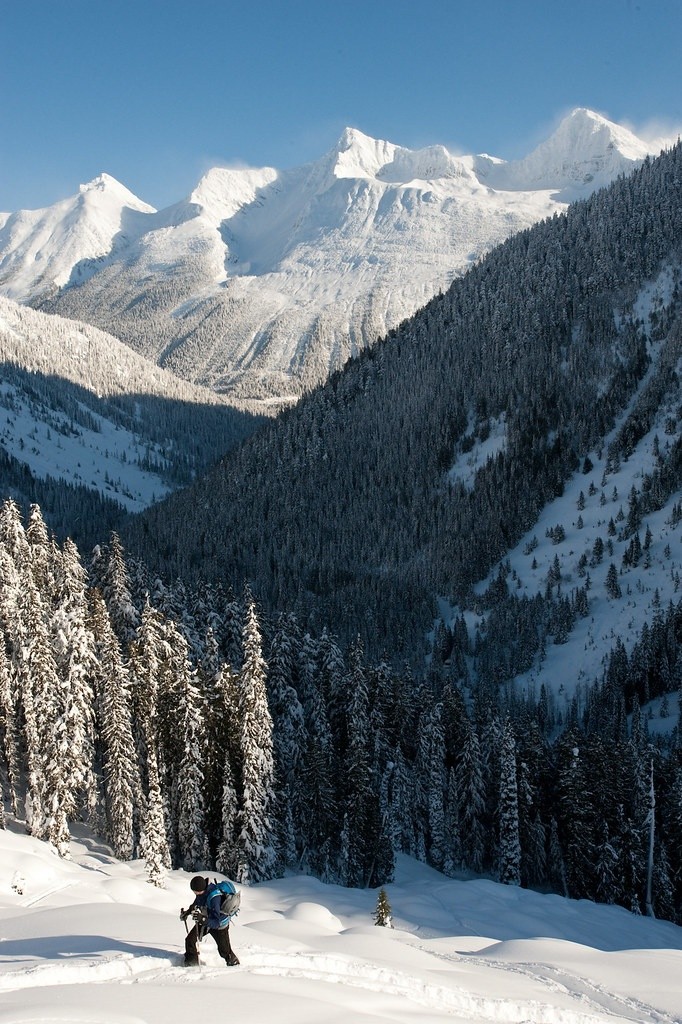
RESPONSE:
[208,880,240,917]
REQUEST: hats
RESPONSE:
[190,876,206,891]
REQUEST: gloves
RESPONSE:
[192,911,208,926]
[180,905,195,921]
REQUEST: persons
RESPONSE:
[180,875,240,967]
